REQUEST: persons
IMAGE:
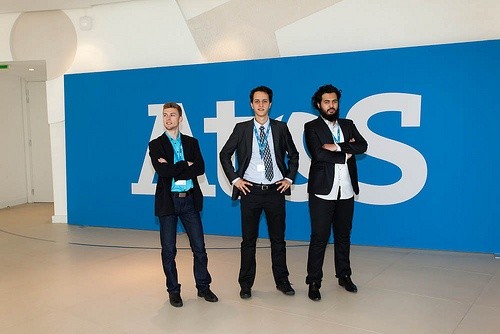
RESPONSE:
[219,86,299,299]
[148,102,218,308]
[303,85,369,301]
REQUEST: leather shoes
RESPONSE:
[338,277,357,293]
[169,293,183,307]
[308,283,321,301]
[240,287,251,299]
[198,288,218,302]
[276,280,295,295]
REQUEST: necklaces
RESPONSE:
[332,124,340,143]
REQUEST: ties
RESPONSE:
[259,126,274,182]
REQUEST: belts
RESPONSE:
[245,184,279,190]
[171,189,194,198]
